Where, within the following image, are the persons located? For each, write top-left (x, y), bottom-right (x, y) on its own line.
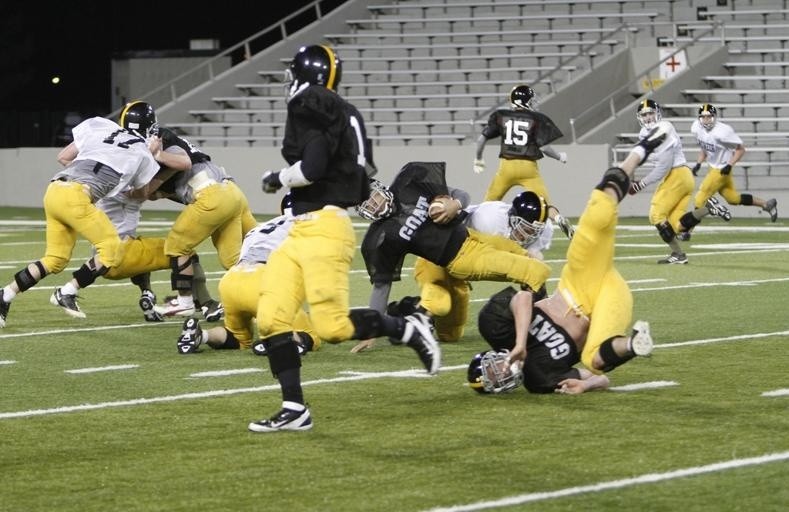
top-left (627, 97), bottom-right (731, 266)
top-left (248, 44), bottom-right (440, 433)
top-left (0, 101), bottom-right (258, 329)
top-left (350, 160), bottom-right (552, 354)
top-left (390, 193), bottom-right (574, 347)
top-left (468, 121), bottom-right (673, 394)
top-left (675, 104), bottom-right (778, 242)
top-left (176, 195), bottom-right (321, 354)
top-left (472, 85), bottom-right (577, 240)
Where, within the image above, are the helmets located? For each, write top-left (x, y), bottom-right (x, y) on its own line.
top-left (635, 99), bottom-right (662, 130)
top-left (697, 104), bottom-right (717, 131)
top-left (353, 179), bottom-right (394, 222)
top-left (506, 191), bottom-right (548, 248)
top-left (280, 191), bottom-right (292, 215)
top-left (509, 85), bottom-right (540, 112)
top-left (467, 347), bottom-right (524, 394)
top-left (281, 45), bottom-right (342, 106)
top-left (117, 99), bottom-right (159, 141)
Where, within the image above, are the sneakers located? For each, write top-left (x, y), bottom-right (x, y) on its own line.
top-left (632, 124), bottom-right (670, 166)
top-left (396, 313), bottom-right (442, 375)
top-left (0, 287), bottom-right (11, 327)
top-left (253, 340), bottom-right (308, 357)
top-left (707, 197), bottom-right (731, 221)
top-left (139, 289), bottom-right (223, 355)
top-left (630, 320), bottom-right (653, 357)
top-left (558, 216), bottom-right (573, 238)
top-left (248, 400), bottom-right (313, 433)
top-left (50, 288), bottom-right (85, 317)
top-left (763, 198), bottom-right (777, 222)
top-left (657, 228), bottom-right (692, 265)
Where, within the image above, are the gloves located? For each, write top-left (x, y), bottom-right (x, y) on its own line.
top-left (720, 165), bottom-right (732, 175)
top-left (558, 152), bottom-right (567, 163)
top-left (693, 164), bottom-right (701, 176)
top-left (628, 182), bottom-right (641, 195)
top-left (473, 159), bottom-right (485, 173)
top-left (262, 170), bottom-right (282, 193)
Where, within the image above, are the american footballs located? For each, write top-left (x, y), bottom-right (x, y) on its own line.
top-left (429, 196), bottom-right (457, 223)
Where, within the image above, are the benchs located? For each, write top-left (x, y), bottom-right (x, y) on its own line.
top-left (165, 0), bottom-right (789, 190)
